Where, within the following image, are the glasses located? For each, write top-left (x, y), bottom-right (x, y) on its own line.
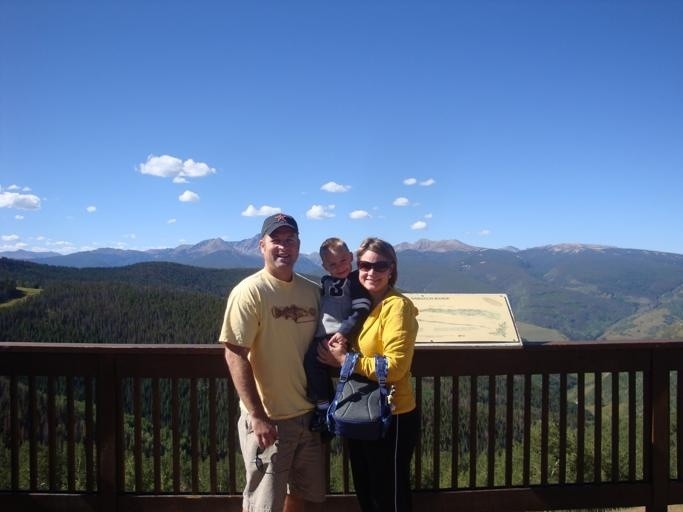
top-left (255, 438), bottom-right (289, 476)
top-left (357, 261), bottom-right (393, 273)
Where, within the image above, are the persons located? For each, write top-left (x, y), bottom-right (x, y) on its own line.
top-left (219, 213), bottom-right (328, 512)
top-left (316, 235), bottom-right (419, 512)
top-left (302, 237), bottom-right (374, 431)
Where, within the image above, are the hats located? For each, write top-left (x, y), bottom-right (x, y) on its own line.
top-left (262, 213), bottom-right (298, 236)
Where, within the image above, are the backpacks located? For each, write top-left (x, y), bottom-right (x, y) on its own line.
top-left (325, 352), bottom-right (393, 441)
